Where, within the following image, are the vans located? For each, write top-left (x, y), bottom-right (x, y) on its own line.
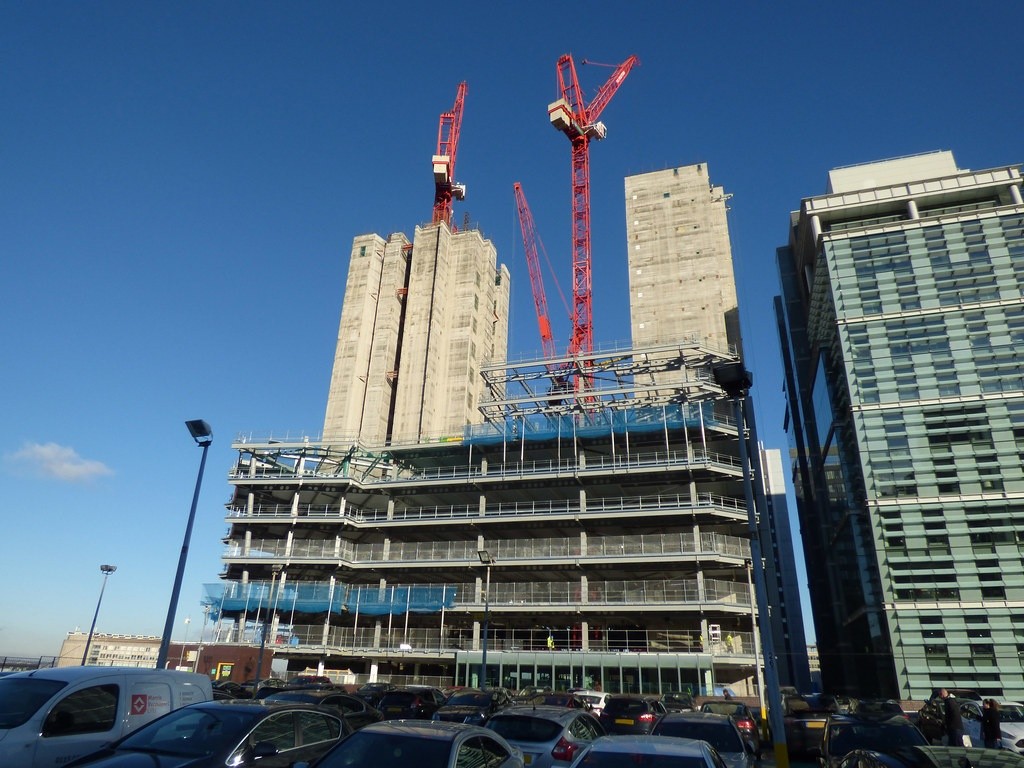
top-left (0, 665), bottom-right (213, 768)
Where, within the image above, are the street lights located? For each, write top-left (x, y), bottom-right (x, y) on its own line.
top-left (476, 550), bottom-right (496, 692)
top-left (710, 362), bottom-right (790, 768)
top-left (156, 419), bottom-right (213, 669)
top-left (81, 564), bottom-right (118, 666)
top-left (252, 564), bottom-right (284, 695)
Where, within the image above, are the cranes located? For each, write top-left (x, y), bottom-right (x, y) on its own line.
top-left (511, 53), bottom-right (642, 427)
top-left (430, 80), bottom-right (468, 229)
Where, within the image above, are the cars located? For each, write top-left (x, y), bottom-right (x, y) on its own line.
top-left (766, 688), bottom-right (1024, 768)
top-left (57, 674), bottom-right (770, 768)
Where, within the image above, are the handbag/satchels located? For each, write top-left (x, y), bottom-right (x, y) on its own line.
top-left (962, 734), bottom-right (973, 748)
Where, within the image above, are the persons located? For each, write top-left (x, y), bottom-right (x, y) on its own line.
top-left (939, 689), bottom-right (964, 747)
top-left (980, 698), bottom-right (1002, 749)
top-left (594, 681), bottom-right (601, 692)
top-left (915, 718), bottom-right (943, 745)
top-left (723, 689), bottom-right (731, 700)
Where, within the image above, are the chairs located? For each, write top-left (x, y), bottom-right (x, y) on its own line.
top-left (207, 715), bottom-right (244, 747)
top-left (704, 706), bottom-right (713, 713)
top-left (735, 707), bottom-right (744, 715)
